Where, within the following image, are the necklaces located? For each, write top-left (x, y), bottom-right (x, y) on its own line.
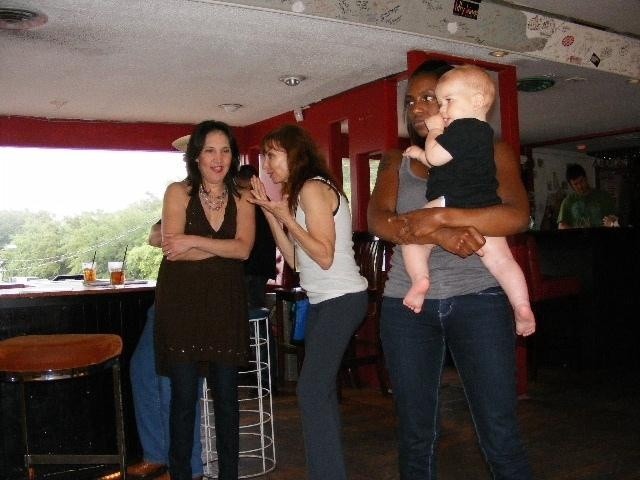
top-left (199, 185), bottom-right (228, 211)
top-left (424, 166), bottom-right (431, 176)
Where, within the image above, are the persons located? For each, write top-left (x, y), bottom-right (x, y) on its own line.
top-left (238, 163), bottom-right (271, 313)
top-left (246, 123), bottom-right (369, 480)
top-left (126, 132), bottom-right (202, 480)
top-left (366, 60), bottom-right (536, 480)
top-left (401, 65), bottom-right (538, 338)
top-left (157, 118), bottom-right (257, 480)
top-left (555, 164), bottom-right (616, 230)
top-left (601, 213), bottom-right (640, 228)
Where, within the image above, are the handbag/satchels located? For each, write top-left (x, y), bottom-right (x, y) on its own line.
top-left (289, 299), bottom-right (309, 347)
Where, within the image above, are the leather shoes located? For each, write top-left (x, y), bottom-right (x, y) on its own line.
top-left (126, 460), bottom-right (171, 480)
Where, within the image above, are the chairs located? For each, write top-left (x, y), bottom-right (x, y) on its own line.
top-left (274, 231), bottom-right (383, 406)
top-left (519, 235), bottom-right (583, 383)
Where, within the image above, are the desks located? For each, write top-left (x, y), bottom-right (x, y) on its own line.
top-left (1, 280), bottom-right (156, 478)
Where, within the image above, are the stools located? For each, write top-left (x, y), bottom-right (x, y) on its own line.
top-left (0, 332), bottom-right (126, 479)
top-left (196, 302), bottom-right (278, 479)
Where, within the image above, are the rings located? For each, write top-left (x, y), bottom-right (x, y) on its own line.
top-left (167, 252), bottom-right (172, 255)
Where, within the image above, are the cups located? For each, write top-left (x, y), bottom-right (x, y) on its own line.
top-left (108, 261), bottom-right (123, 285)
top-left (82, 263), bottom-right (97, 284)
